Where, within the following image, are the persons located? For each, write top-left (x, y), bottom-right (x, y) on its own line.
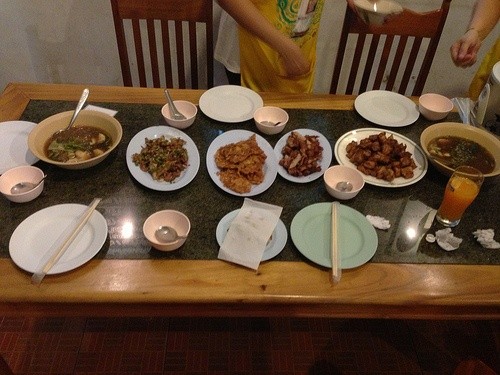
top-left (451, 0), bottom-right (500, 97)
top-left (214, 0), bottom-right (376, 91)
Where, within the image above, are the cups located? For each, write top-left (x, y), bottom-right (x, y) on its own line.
top-left (435, 167), bottom-right (484, 227)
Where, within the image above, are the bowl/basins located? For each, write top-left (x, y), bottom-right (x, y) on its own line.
top-left (421, 123), bottom-right (500, 177)
top-left (161, 100), bottom-right (197, 129)
top-left (418, 93), bottom-right (453, 120)
top-left (142, 209), bottom-right (190, 252)
top-left (0, 166), bottom-right (44, 203)
top-left (324, 164), bottom-right (365, 200)
top-left (28, 109), bottom-right (122, 168)
top-left (254, 105), bottom-right (288, 135)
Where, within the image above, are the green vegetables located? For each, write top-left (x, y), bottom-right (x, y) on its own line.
top-left (48, 131), bottom-right (112, 160)
top-left (149, 147), bottom-right (188, 173)
top-left (448, 138), bottom-right (482, 168)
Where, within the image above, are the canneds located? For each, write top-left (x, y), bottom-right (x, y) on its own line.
top-left (470, 60), bottom-right (500, 139)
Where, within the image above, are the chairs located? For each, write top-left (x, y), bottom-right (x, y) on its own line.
top-left (110, 0), bottom-right (214, 90)
top-left (328, 0), bottom-right (452, 96)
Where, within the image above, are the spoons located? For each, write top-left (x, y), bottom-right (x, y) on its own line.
top-left (164, 89), bottom-right (186, 120)
top-left (155, 226), bottom-right (187, 242)
top-left (261, 120), bottom-right (281, 126)
top-left (10, 175), bottom-right (47, 194)
top-left (336, 181), bottom-right (353, 192)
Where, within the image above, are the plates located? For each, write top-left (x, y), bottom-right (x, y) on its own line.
top-left (8, 203), bottom-right (108, 274)
top-left (126, 125), bottom-right (200, 191)
top-left (353, 0), bottom-right (402, 23)
top-left (0, 120), bottom-right (40, 174)
top-left (199, 85), bottom-right (263, 123)
top-left (206, 90), bottom-right (428, 269)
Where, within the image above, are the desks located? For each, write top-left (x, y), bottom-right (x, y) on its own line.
top-left (0, 82), bottom-right (500, 375)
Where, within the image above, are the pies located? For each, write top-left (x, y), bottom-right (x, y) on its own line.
top-left (215, 133), bottom-right (267, 193)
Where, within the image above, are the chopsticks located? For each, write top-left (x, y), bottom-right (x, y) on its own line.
top-left (331, 202), bottom-right (338, 277)
top-left (42, 198), bottom-right (100, 272)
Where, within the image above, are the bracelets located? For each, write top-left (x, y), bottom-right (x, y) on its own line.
top-left (464, 28), bottom-right (480, 37)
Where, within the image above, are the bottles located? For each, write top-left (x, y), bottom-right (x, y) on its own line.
top-left (472, 61), bottom-right (500, 134)
